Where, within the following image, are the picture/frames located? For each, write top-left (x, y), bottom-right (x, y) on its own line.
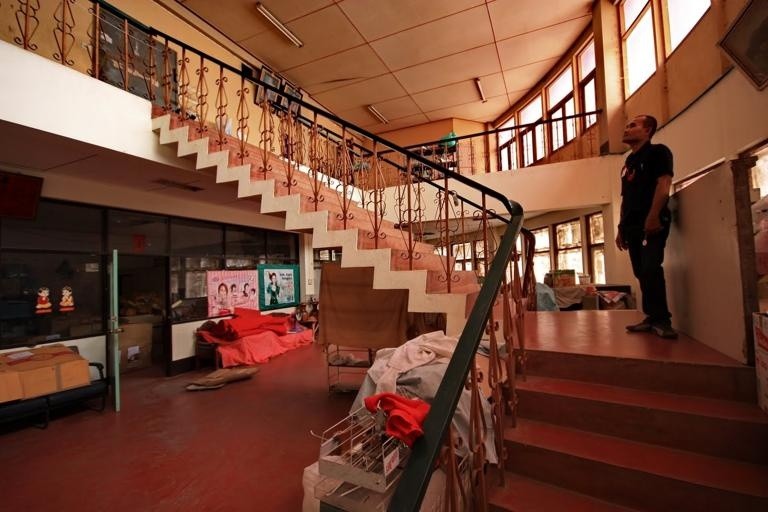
top-left (255, 66), bottom-right (303, 125)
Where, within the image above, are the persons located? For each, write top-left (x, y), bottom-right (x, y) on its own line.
top-left (615, 114), bottom-right (679, 339)
top-left (267, 273), bottom-right (280, 305)
top-left (213, 282), bottom-right (257, 313)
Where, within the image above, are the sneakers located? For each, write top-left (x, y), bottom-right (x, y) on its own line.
top-left (650, 324), bottom-right (678, 339)
top-left (626, 322), bottom-right (654, 332)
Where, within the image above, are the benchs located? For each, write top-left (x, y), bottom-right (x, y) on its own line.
top-left (1, 345), bottom-right (108, 430)
